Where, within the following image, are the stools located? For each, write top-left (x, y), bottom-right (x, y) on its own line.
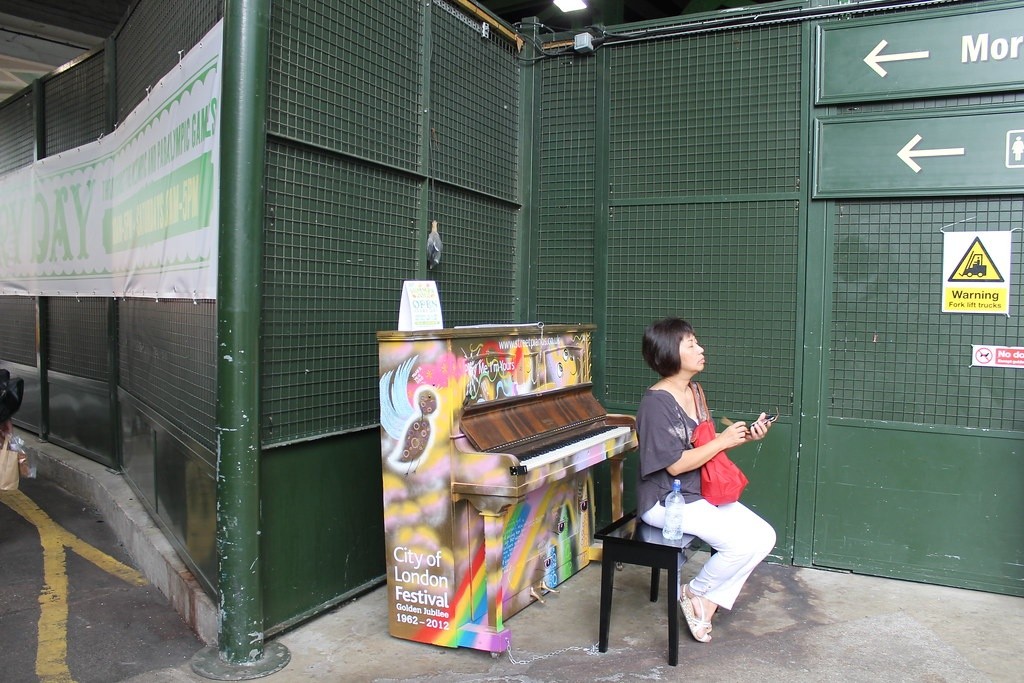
top-left (593, 509), bottom-right (718, 666)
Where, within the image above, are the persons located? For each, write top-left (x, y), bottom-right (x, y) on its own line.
top-left (0, 368), bottom-right (24, 450)
top-left (634, 316), bottom-right (776, 641)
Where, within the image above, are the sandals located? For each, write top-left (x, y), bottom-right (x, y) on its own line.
top-left (680, 583), bottom-right (712, 643)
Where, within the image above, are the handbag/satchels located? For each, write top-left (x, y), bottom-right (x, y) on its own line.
top-left (687, 382), bottom-right (748, 507)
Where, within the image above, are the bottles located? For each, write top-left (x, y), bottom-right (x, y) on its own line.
top-left (663, 480), bottom-right (685, 540)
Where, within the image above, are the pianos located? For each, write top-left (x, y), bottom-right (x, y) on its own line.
top-left (375, 321), bottom-right (639, 660)
